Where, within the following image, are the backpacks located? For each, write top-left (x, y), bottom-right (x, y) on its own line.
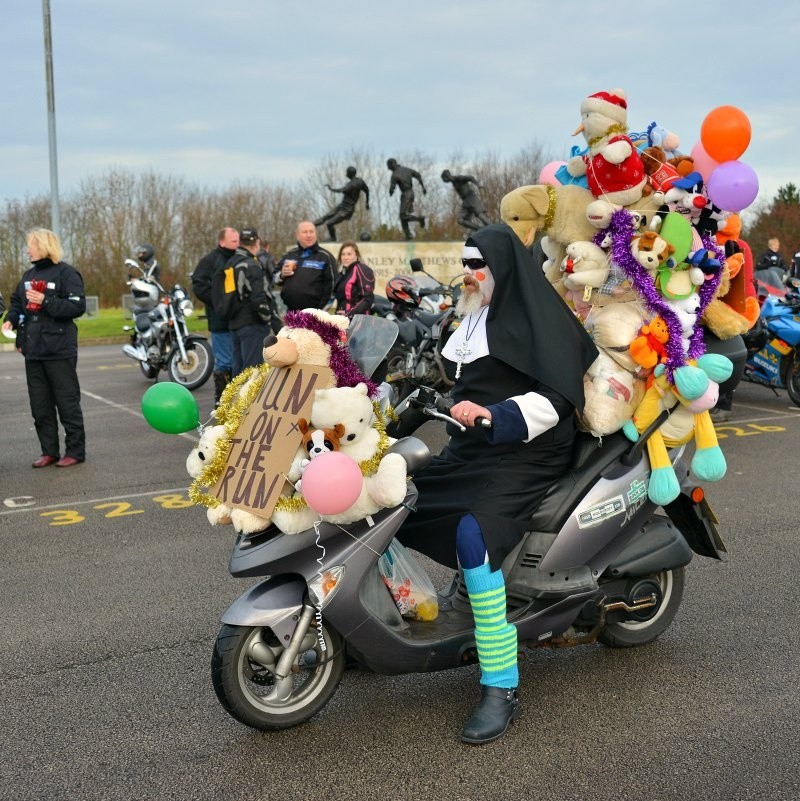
top-left (212, 255), bottom-right (250, 320)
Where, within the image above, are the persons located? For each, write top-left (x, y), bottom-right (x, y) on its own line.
top-left (311, 166), bottom-right (371, 243)
top-left (192, 228), bottom-right (241, 411)
top-left (386, 158), bottom-right (427, 241)
top-left (389, 222), bottom-right (584, 738)
top-left (441, 169), bottom-right (491, 231)
top-left (757, 239), bottom-right (788, 269)
top-left (259, 238), bottom-right (276, 285)
top-left (134, 243), bottom-right (162, 298)
top-left (270, 223), bottom-right (339, 315)
top-left (226, 227), bottom-right (272, 380)
top-left (1, 226), bottom-right (88, 467)
top-left (334, 241), bottom-right (375, 319)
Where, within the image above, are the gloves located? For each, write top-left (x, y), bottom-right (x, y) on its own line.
top-left (26, 278), bottom-right (47, 309)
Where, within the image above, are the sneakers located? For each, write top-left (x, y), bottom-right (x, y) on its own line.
top-left (56, 456), bottom-right (77, 466)
top-left (31, 454), bottom-right (60, 468)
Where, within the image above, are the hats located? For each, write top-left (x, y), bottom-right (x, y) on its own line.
top-left (240, 228), bottom-right (258, 240)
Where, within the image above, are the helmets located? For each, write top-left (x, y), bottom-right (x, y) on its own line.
top-left (137, 244), bottom-right (155, 261)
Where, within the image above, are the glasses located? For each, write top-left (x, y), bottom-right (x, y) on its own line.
top-left (461, 258), bottom-right (487, 270)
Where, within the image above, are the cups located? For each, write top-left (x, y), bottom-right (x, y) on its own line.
top-left (24, 280), bottom-right (38, 304)
top-left (284, 259), bottom-right (295, 276)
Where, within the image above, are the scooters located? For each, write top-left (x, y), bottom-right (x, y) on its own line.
top-left (320, 255), bottom-right (468, 402)
top-left (208, 310), bottom-right (745, 733)
top-left (747, 260), bottom-right (800, 410)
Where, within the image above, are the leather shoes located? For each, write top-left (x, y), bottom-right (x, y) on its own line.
top-left (460, 685), bottom-right (523, 743)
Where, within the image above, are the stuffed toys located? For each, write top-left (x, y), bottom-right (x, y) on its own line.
top-left (186, 305), bottom-right (409, 538)
top-left (492, 86), bottom-right (760, 508)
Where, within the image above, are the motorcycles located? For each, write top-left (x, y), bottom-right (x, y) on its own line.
top-left (122, 282), bottom-right (216, 391)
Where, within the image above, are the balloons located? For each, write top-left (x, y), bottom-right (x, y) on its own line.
top-left (688, 141), bottom-right (718, 186)
top-left (702, 105), bottom-right (752, 163)
top-left (298, 452), bottom-right (367, 521)
top-left (142, 381), bottom-right (203, 437)
top-left (537, 161), bottom-right (573, 188)
top-left (708, 164), bottom-right (758, 214)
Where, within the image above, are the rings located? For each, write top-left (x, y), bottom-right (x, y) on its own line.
top-left (462, 412), bottom-right (468, 417)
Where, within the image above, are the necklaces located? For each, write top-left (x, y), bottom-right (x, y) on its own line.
top-left (454, 305), bottom-right (487, 379)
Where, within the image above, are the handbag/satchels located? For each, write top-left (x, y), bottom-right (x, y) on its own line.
top-left (376, 540), bottom-right (439, 622)
